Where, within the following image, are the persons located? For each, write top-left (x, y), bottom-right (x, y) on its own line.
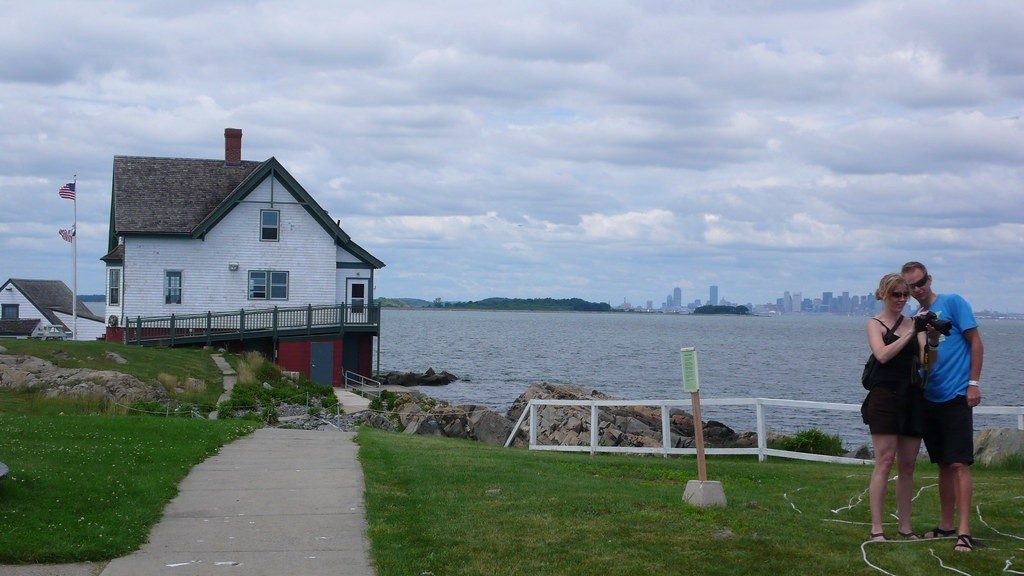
top-left (900, 261), bottom-right (984, 553)
top-left (860, 273), bottom-right (943, 542)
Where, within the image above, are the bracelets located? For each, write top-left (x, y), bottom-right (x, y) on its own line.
top-left (929, 342), bottom-right (939, 351)
top-left (969, 380), bottom-right (980, 387)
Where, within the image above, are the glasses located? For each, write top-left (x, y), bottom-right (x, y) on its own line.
top-left (889, 291), bottom-right (909, 298)
top-left (907, 274), bottom-right (929, 290)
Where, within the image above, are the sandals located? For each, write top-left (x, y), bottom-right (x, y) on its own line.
top-left (871, 532), bottom-right (888, 541)
top-left (898, 531), bottom-right (921, 540)
top-left (921, 527), bottom-right (956, 538)
top-left (953, 535), bottom-right (972, 552)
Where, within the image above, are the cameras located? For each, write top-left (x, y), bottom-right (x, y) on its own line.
top-left (914, 310), bottom-right (953, 337)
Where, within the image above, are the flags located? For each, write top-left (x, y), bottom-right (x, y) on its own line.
top-left (58, 224), bottom-right (76, 244)
top-left (59, 183), bottom-right (76, 200)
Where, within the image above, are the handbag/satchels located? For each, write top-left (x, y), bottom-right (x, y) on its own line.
top-left (862, 353), bottom-right (886, 390)
